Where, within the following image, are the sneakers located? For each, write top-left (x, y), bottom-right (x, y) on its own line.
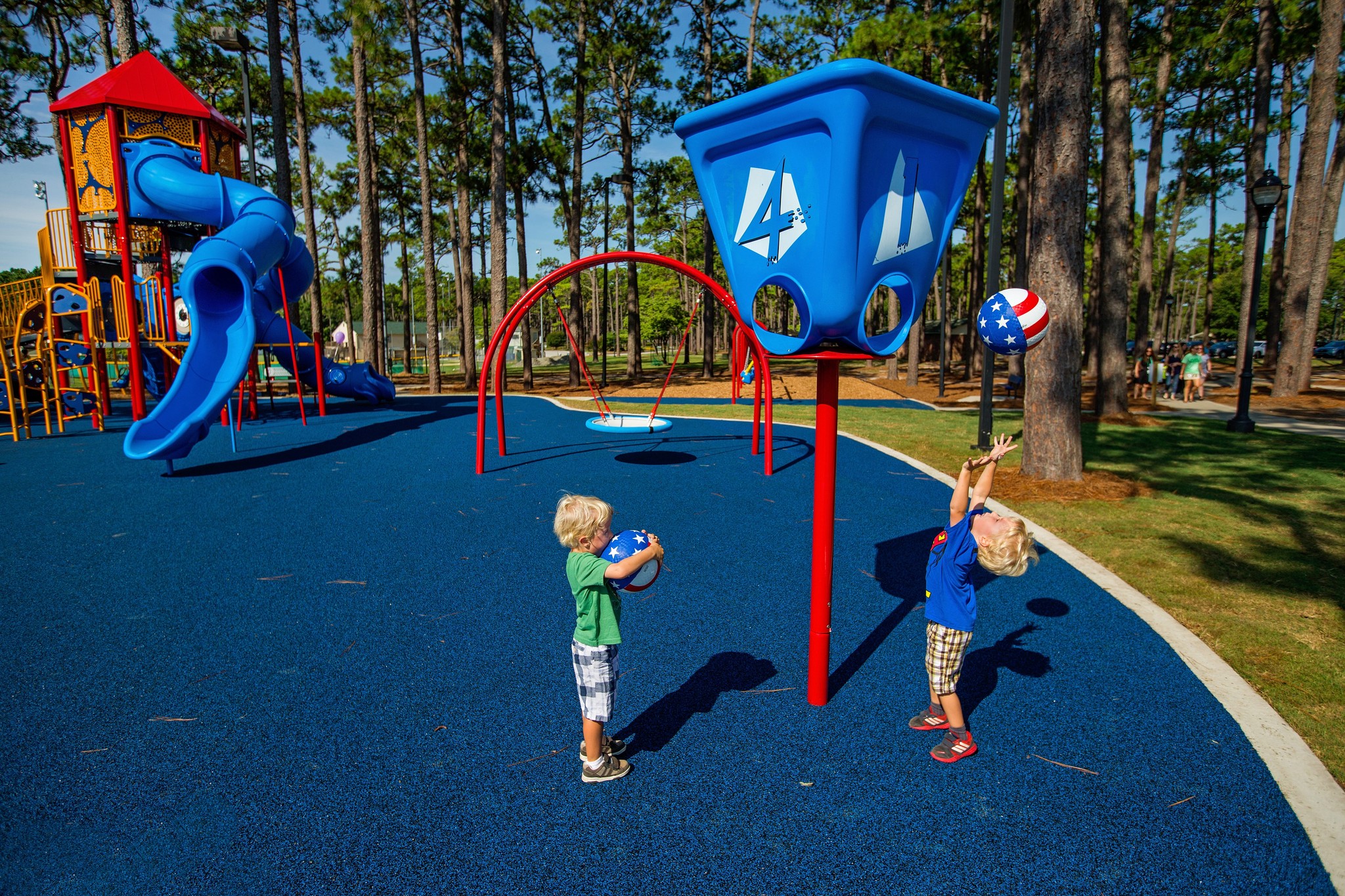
top-left (930, 731), bottom-right (977, 762)
top-left (908, 706), bottom-right (950, 730)
top-left (580, 735), bottom-right (627, 761)
top-left (581, 752), bottom-right (630, 783)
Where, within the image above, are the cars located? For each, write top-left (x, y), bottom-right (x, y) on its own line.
top-left (1125, 341), bottom-right (1134, 355)
top-left (1208, 341), bottom-right (1281, 359)
top-left (1313, 341), bottom-right (1345, 359)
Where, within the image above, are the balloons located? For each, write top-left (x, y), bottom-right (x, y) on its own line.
top-left (335, 332), bottom-right (345, 344)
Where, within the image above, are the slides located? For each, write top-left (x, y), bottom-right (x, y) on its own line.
top-left (250, 232), bottom-right (396, 400)
top-left (123, 145), bottom-right (296, 461)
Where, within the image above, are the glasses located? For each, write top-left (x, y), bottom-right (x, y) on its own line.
top-left (1193, 348), bottom-right (1199, 350)
top-left (1172, 346), bottom-right (1178, 348)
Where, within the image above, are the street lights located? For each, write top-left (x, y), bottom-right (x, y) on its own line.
top-left (536, 248), bottom-right (544, 359)
top-left (444, 272), bottom-right (453, 355)
top-left (33, 180), bottom-right (54, 256)
top-left (1227, 163), bottom-right (1292, 432)
top-left (438, 283), bottom-right (446, 348)
top-left (1162, 294), bottom-right (1174, 359)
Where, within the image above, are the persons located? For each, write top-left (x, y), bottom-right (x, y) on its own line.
top-left (553, 494), bottom-right (664, 783)
top-left (908, 434), bottom-right (1040, 763)
top-left (1131, 347), bottom-right (1154, 399)
top-left (1163, 343), bottom-right (1212, 403)
top-left (21, 349), bottom-right (30, 360)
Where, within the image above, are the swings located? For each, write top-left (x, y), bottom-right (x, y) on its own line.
top-left (547, 284), bottom-right (705, 436)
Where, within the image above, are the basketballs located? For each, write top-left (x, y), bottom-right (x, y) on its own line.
top-left (976, 289), bottom-right (1049, 356)
top-left (597, 530), bottom-right (661, 595)
